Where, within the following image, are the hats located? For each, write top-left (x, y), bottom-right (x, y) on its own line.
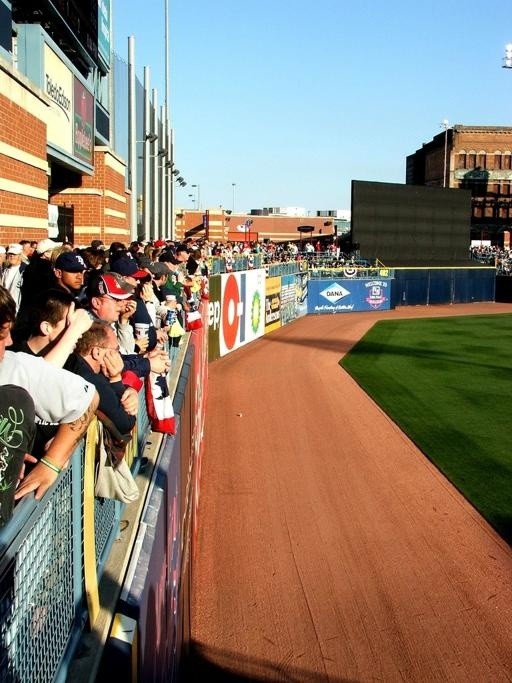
top-left (0, 240), bottom-right (195, 300)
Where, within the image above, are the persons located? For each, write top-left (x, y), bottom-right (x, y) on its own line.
top-left (0, 239), bottom-right (364, 683)
top-left (471, 245), bottom-right (512, 276)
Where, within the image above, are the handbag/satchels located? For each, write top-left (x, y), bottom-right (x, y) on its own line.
top-left (186, 311), bottom-right (202, 331)
top-left (145, 370), bottom-right (176, 436)
top-left (95, 446), bottom-right (140, 503)
top-left (168, 318), bottom-right (185, 338)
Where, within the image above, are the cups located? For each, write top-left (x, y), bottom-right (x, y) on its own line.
top-left (166, 294), bottom-right (176, 304)
top-left (166, 308), bottom-right (176, 325)
top-left (135, 321), bottom-right (149, 337)
